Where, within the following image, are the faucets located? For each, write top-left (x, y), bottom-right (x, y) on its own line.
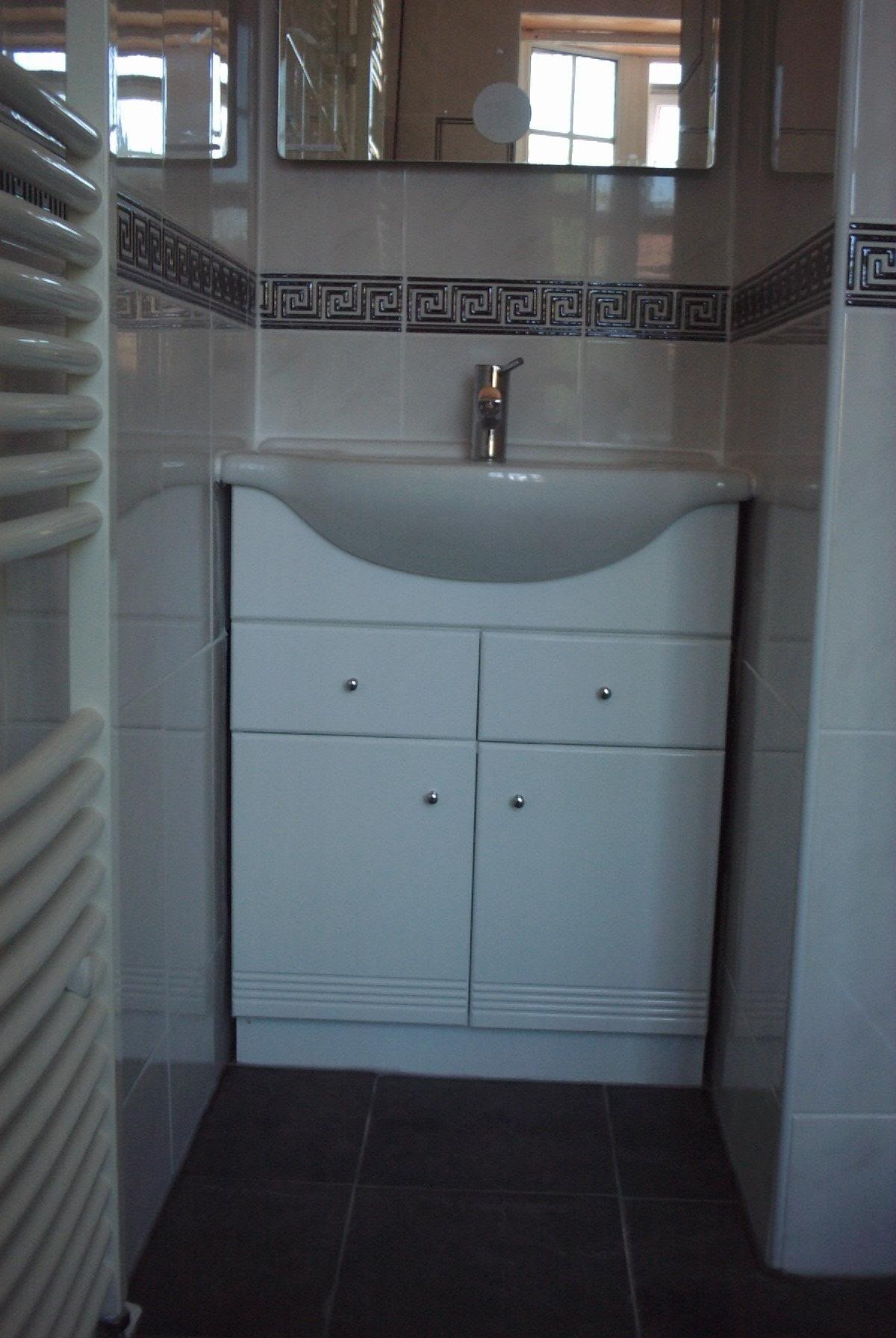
top-left (469, 357), bottom-right (526, 462)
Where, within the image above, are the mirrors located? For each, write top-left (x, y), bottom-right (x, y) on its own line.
top-left (273, 0), bottom-right (722, 173)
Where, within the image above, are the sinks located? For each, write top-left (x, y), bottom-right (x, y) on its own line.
top-left (222, 435), bottom-right (704, 480)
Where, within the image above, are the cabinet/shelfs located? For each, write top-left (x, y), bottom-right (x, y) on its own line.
top-left (226, 484), bottom-right (729, 1087)
